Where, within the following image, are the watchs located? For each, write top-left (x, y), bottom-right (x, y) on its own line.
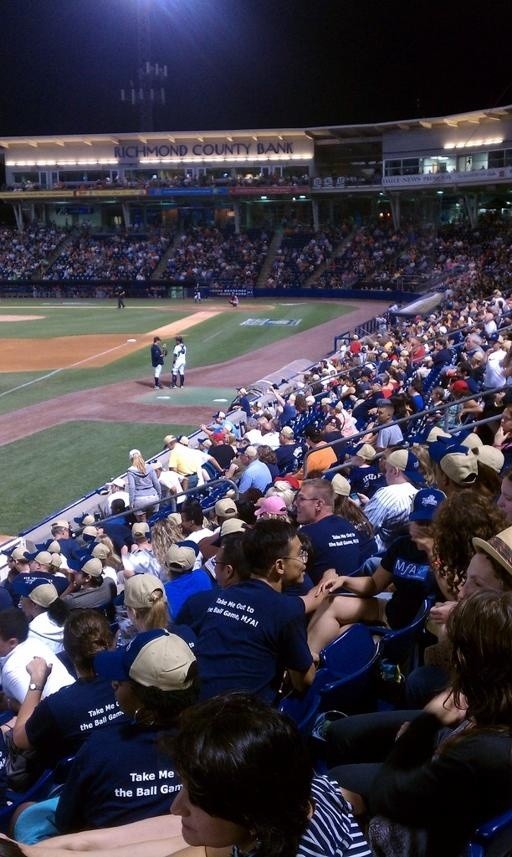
top-left (28, 684), bottom-right (43, 691)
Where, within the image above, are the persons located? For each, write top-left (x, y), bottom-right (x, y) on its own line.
top-left (253, 496), bottom-right (285, 524)
top-left (333, 590), bottom-right (512, 820)
top-left (168, 335), bottom-right (187, 388)
top-left (164, 513), bottom-right (185, 528)
top-left (145, 519), bottom-right (182, 576)
top-left (273, 223), bottom-right (461, 297)
top-left (46, 516), bottom-right (81, 568)
top-left (406, 428), bottom-right (449, 487)
top-left (268, 480), bottom-right (295, 517)
top-left (84, 522), bottom-right (136, 553)
top-left (37, 536), bottom-right (63, 559)
top-left (73, 223), bottom-right (273, 290)
top-left (302, 481), bottom-right (442, 671)
top-left (173, 432), bottom-right (224, 485)
top-left (257, 445), bottom-right (278, 481)
top-left (158, 691), bottom-right (376, 857)
top-left (10, 628), bottom-right (207, 848)
top-left (161, 433), bottom-right (200, 490)
top-left (11, 548), bottom-right (66, 600)
top-left (101, 480), bottom-right (130, 516)
top-left (185, 521), bottom-right (334, 714)
top-left (126, 446), bottom-right (162, 524)
top-left (0, 224), bottom-right (73, 291)
top-left (316, 524), bottom-right (512, 745)
top-left (82, 542), bottom-right (118, 591)
top-left (14, 610), bottom-right (126, 766)
top-left (288, 482), bottom-right (362, 581)
top-left (209, 496), bottom-right (236, 529)
top-left (68, 511), bottom-right (99, 530)
top-left (461, 219), bottom-right (512, 292)
top-left (235, 445), bottom-right (272, 499)
top-left (193, 294), bottom-right (512, 452)
top-left (1, 543), bottom-right (30, 581)
top-left (17, 573), bottom-right (73, 653)
top-left (114, 576), bottom-right (201, 682)
top-left (78, 523), bottom-right (98, 550)
top-left (423, 490), bottom-right (506, 595)
top-left (116, 285), bottom-right (127, 309)
top-left (498, 468), bottom-right (512, 528)
top-left (196, 520), bottom-right (250, 558)
top-left (10, 172), bottom-right (361, 193)
top-left (158, 549), bottom-right (214, 625)
top-left (175, 499), bottom-right (212, 548)
top-left (464, 441), bottom-right (503, 499)
top-left (94, 534), bottom-right (122, 569)
top-left (101, 497), bottom-right (134, 530)
top-left (328, 474), bottom-right (375, 536)
top-left (361, 445), bottom-right (420, 553)
top-left (120, 523), bottom-right (161, 576)
top-left (0, 607), bottom-right (78, 738)
top-left (59, 550), bottom-right (118, 631)
top-left (338, 440), bottom-right (377, 493)
top-left (432, 449), bottom-right (474, 492)
top-left (46, 551), bottom-right (75, 595)
top-left (147, 456), bottom-right (189, 507)
top-left (172, 534), bottom-right (247, 644)
top-left (151, 336), bottom-right (166, 389)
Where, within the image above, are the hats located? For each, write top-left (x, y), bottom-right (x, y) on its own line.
top-left (282, 379), bottom-right (288, 383)
top-left (34, 551), bottom-right (52, 564)
top-left (112, 574), bottom-right (168, 608)
top-left (215, 497), bottom-right (238, 518)
top-left (281, 426), bottom-right (294, 438)
top-left (73, 513), bottom-right (95, 526)
top-left (212, 411), bottom-right (226, 418)
top-left (161, 435), bottom-right (176, 449)
top-left (328, 400), bottom-right (343, 411)
top-left (471, 527), bottom-right (512, 574)
top-left (105, 478), bottom-right (126, 489)
top-left (344, 441), bottom-right (376, 461)
top-left (386, 449), bottom-right (426, 483)
top-left (154, 337), bottom-right (161, 341)
top-left (92, 628), bottom-right (197, 690)
top-left (486, 332), bottom-right (503, 344)
top-left (321, 471), bottom-right (351, 498)
top-left (169, 511), bottom-right (183, 525)
top-left (237, 446), bottom-right (258, 458)
top-left (82, 525), bottom-right (97, 538)
top-left (50, 519), bottom-right (73, 537)
top-left (302, 427), bottom-right (320, 437)
top-left (409, 488), bottom-right (448, 523)
top-left (164, 540), bottom-right (200, 574)
top-left (35, 539), bottom-right (60, 554)
top-left (177, 434), bottom-right (190, 446)
top-left (322, 416), bottom-right (341, 426)
top-left (198, 438), bottom-right (213, 448)
top-left (254, 496), bottom-right (288, 517)
top-left (149, 459), bottom-right (162, 470)
top-left (449, 380), bottom-right (469, 391)
top-left (220, 518), bottom-right (247, 536)
top-left (2, 545), bottom-right (16, 559)
top-left (473, 442), bottom-right (506, 474)
top-left (11, 572), bottom-right (59, 608)
top-left (236, 386), bottom-right (246, 395)
top-left (76, 542), bottom-right (110, 560)
top-left (131, 522), bottom-right (150, 539)
top-left (270, 384), bottom-right (279, 390)
top-left (309, 368), bottom-right (318, 374)
top-left (437, 427), bottom-right (483, 451)
top-left (235, 438), bottom-right (250, 448)
top-left (128, 449), bottom-right (141, 457)
top-left (24, 549), bottom-right (37, 560)
top-left (429, 442), bottom-right (478, 487)
top-left (174, 336), bottom-right (182, 340)
top-left (49, 553), bottom-right (62, 566)
top-left (67, 556), bottom-right (103, 577)
top-left (9, 548), bottom-right (29, 561)
top-left (409, 425), bottom-right (446, 444)
top-left (466, 351), bottom-right (484, 363)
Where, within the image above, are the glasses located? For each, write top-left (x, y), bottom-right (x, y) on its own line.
top-left (299, 496), bottom-right (319, 502)
top-left (282, 550), bottom-right (309, 563)
top-left (211, 558), bottom-right (225, 565)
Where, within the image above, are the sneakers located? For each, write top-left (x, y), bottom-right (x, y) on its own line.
top-left (153, 384), bottom-right (163, 389)
top-left (180, 385), bottom-right (184, 389)
top-left (168, 384), bottom-right (176, 388)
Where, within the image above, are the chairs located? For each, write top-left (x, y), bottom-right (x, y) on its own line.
top-left (276, 686), bottom-right (321, 732)
top-left (315, 623), bottom-right (385, 700)
top-left (146, 503), bottom-right (172, 528)
top-left (419, 354), bottom-right (455, 406)
top-left (449, 837), bottom-right (489, 857)
top-left (481, 802), bottom-right (512, 857)
top-left (282, 399), bottom-right (324, 445)
top-left (367, 595), bottom-right (436, 658)
top-left (192, 465), bottom-right (244, 515)
